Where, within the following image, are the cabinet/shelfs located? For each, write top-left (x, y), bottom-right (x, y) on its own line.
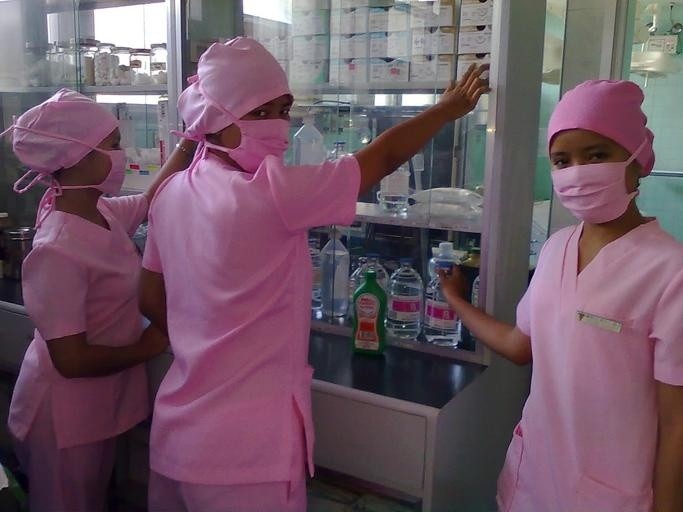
top-left (0, 0), bottom-right (174, 511)
top-left (175, 1), bottom-right (546, 512)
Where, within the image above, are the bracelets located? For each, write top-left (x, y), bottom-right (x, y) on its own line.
top-left (175, 144), bottom-right (192, 156)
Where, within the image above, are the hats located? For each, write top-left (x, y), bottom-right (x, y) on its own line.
top-left (177, 37), bottom-right (293, 138)
top-left (13, 88), bottom-right (120, 175)
top-left (548, 80), bottom-right (656, 177)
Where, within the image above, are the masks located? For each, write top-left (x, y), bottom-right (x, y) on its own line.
top-left (551, 138), bottom-right (651, 223)
top-left (0, 115), bottom-right (126, 230)
top-left (168, 75), bottom-right (290, 175)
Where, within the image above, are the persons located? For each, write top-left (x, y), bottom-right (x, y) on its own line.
top-left (0, 87), bottom-right (197, 511)
top-left (137, 37), bottom-right (491, 511)
top-left (436, 80), bottom-right (683, 511)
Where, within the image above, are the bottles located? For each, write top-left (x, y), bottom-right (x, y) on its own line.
top-left (319, 229), bottom-right (349, 317)
top-left (380, 161), bottom-right (407, 211)
top-left (424, 242), bottom-right (480, 347)
top-left (292, 113), bottom-right (346, 169)
top-left (386, 260), bottom-right (423, 338)
top-left (350, 253), bottom-right (389, 312)
top-left (306, 239), bottom-right (321, 319)
